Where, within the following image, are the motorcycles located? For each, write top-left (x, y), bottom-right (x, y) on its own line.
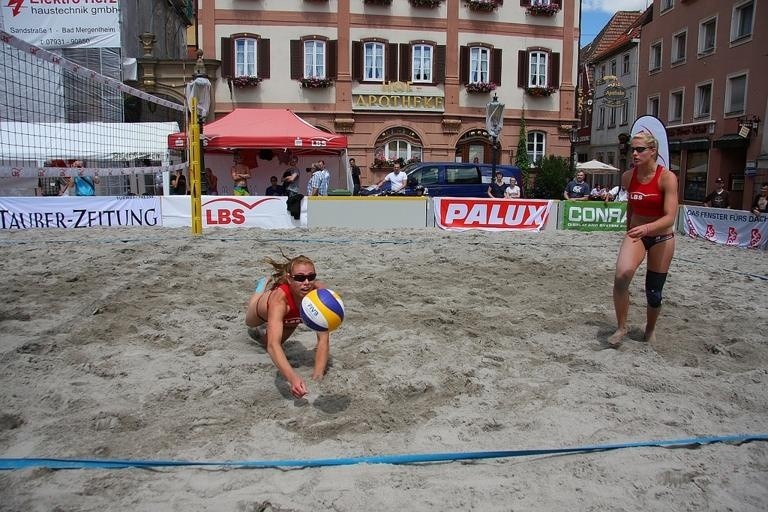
top-left (378, 188), bottom-right (397, 196)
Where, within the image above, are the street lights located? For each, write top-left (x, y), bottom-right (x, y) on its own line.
top-left (486, 93), bottom-right (505, 184)
top-left (184, 50), bottom-right (211, 195)
top-left (568, 125), bottom-right (580, 182)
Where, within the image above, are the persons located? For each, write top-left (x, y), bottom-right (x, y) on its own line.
top-left (36, 160), bottom-right (68, 196)
top-left (246, 244), bottom-right (330, 399)
top-left (487, 172), bottom-right (505, 198)
top-left (606, 128), bottom-right (678, 347)
top-left (349, 158), bottom-right (364, 196)
top-left (563, 172), bottom-right (590, 202)
top-left (231, 156), bottom-right (251, 196)
top-left (170, 165), bottom-right (186, 194)
top-left (68, 159), bottom-right (100, 196)
top-left (505, 177), bottom-right (520, 199)
top-left (204, 168), bottom-right (218, 194)
top-left (266, 156), bottom-right (330, 196)
top-left (589, 184), bottom-right (627, 202)
top-left (374, 162), bottom-right (406, 195)
top-left (703, 177), bottom-right (732, 209)
top-left (749, 183), bottom-right (768, 216)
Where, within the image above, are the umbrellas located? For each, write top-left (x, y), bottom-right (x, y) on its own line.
top-left (575, 159), bottom-right (621, 190)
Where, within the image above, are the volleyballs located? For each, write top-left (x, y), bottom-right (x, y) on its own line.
top-left (300, 289), bottom-right (344, 331)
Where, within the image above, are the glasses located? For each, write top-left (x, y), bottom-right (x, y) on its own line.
top-left (289, 274), bottom-right (315, 282)
top-left (630, 147), bottom-right (649, 153)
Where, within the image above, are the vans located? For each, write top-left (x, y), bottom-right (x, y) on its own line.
top-left (364, 162), bottom-right (524, 199)
